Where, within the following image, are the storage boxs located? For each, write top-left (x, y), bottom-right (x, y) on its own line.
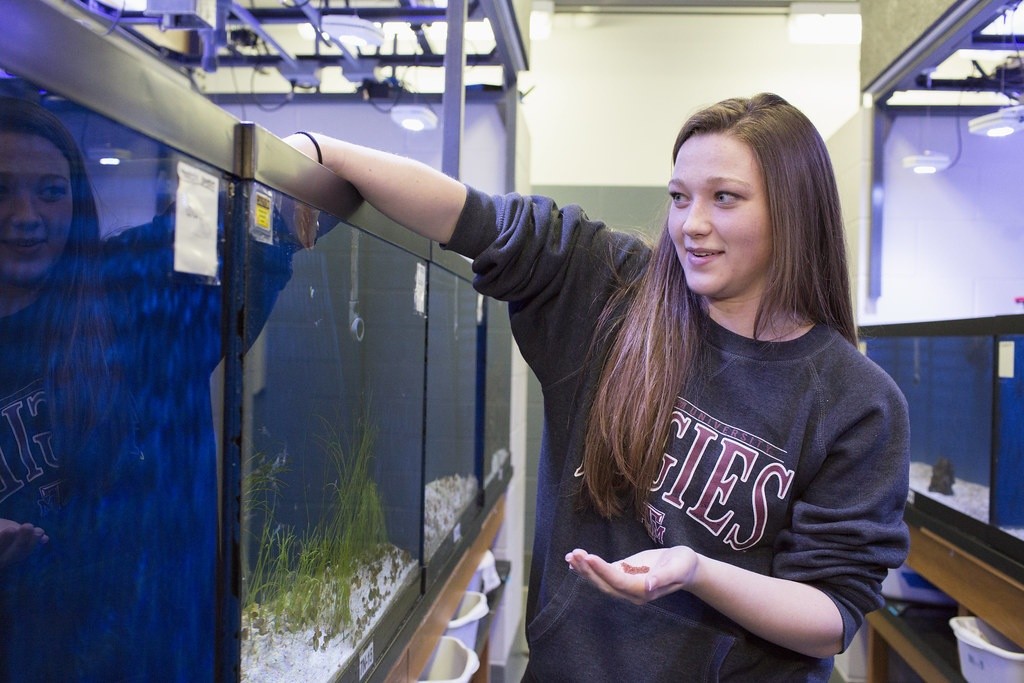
top-left (858, 312), bottom-right (1024, 586)
top-left (0, 1), bottom-right (514, 683)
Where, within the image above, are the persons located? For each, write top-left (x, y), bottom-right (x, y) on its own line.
top-left (280, 92), bottom-right (910, 683)
top-left (0, 95), bottom-right (338, 683)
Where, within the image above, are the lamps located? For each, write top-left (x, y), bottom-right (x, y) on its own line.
top-left (390, 104), bottom-right (439, 132)
top-left (965, 3), bottom-right (1024, 139)
top-left (901, 68), bottom-right (951, 174)
top-left (320, 11), bottom-right (384, 48)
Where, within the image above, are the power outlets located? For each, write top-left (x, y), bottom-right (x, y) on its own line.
top-left (340, 59), bottom-right (377, 82)
top-left (279, 60), bottom-right (323, 86)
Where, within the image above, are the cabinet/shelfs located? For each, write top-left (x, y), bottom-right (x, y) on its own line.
top-left (377, 491), bottom-right (514, 683)
top-left (865, 518), bottom-right (1024, 683)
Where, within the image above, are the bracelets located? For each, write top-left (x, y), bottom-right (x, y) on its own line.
top-left (295, 131), bottom-right (322, 164)
